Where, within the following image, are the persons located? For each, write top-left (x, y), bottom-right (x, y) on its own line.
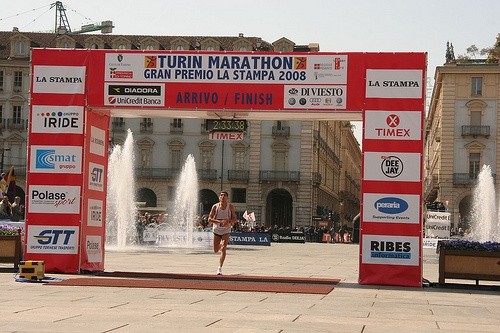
top-left (135, 212), bottom-right (353, 243)
top-left (208, 191), bottom-right (237, 275)
top-left (0, 173), bottom-right (25, 221)
top-left (439, 202), bottom-right (445, 212)
top-left (451, 228), bottom-right (471, 237)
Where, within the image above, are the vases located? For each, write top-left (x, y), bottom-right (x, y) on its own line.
top-left (439, 246), bottom-right (500, 285)
top-left (0, 235), bottom-right (23, 272)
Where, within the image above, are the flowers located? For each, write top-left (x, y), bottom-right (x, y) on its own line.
top-left (444, 239), bottom-right (500, 252)
top-left (0, 224), bottom-right (25, 238)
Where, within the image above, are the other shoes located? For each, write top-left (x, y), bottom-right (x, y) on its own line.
top-left (218, 268), bottom-right (222, 275)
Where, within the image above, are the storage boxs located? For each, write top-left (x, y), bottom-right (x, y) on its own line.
top-left (19, 260), bottom-right (45, 280)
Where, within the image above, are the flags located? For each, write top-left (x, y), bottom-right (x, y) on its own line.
top-left (0, 165), bottom-right (15, 204)
